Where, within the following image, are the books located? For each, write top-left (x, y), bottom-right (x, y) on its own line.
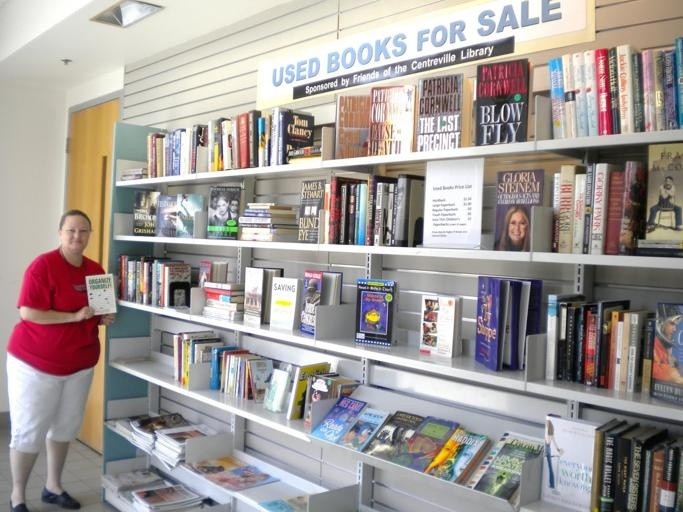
top-left (315, 395), bottom-right (543, 501)
top-left (475, 275), bottom-right (682, 406)
top-left (327, 166), bottom-right (543, 252)
top-left (333, 37), bottom-right (682, 159)
top-left (84, 273), bottom-right (116, 316)
top-left (540, 412), bottom-right (681, 512)
top-left (118, 106), bottom-right (321, 180)
top-left (549, 142), bottom-right (683, 258)
top-left (354, 277), bottom-right (398, 350)
top-left (104, 330), bottom-right (355, 511)
top-left (121, 255), bottom-right (348, 336)
top-left (421, 294), bottom-right (462, 356)
top-left (133, 180), bottom-right (327, 245)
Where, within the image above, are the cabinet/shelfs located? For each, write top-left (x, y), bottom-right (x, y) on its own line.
top-left (101, 63), bottom-right (682, 512)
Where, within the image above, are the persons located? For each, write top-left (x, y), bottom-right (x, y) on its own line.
top-left (2, 210), bottom-right (114, 511)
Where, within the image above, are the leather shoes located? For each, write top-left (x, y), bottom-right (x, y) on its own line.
top-left (9, 500), bottom-right (29, 512)
top-left (42, 487), bottom-right (81, 509)
